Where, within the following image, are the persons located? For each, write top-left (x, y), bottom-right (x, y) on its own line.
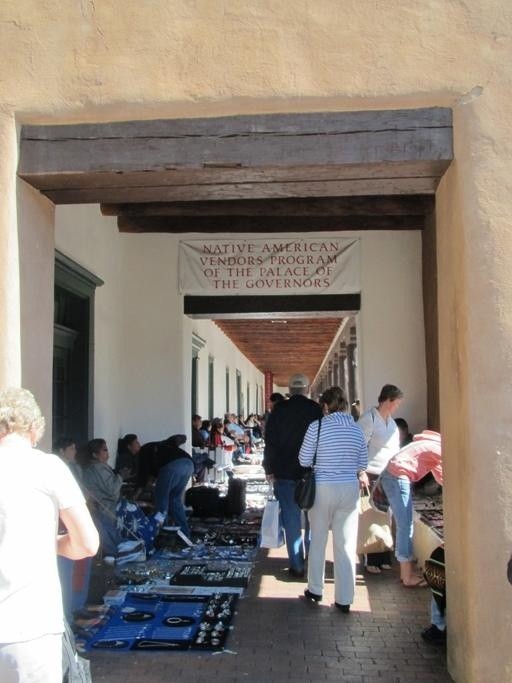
top-left (192, 413), bottom-right (265, 465)
top-left (393, 418), bottom-right (413, 447)
top-left (355, 383), bottom-right (404, 574)
top-left (263, 374), bottom-right (324, 578)
top-left (1, 386), bottom-right (100, 683)
top-left (51, 433), bottom-right (194, 553)
top-left (381, 429), bottom-right (444, 588)
top-left (298, 387), bottom-right (369, 612)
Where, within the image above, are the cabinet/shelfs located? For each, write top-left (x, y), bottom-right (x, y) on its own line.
top-left (407, 492), bottom-right (444, 575)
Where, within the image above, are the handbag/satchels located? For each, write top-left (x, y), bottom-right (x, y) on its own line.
top-left (357, 496), bottom-right (393, 554)
top-left (261, 500), bottom-right (284, 548)
top-left (297, 470), bottom-right (315, 509)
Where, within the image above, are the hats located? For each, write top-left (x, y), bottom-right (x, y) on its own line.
top-left (289, 374), bottom-right (309, 388)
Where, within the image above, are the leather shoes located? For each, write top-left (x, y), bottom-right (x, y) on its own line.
top-left (304, 590), bottom-right (321, 601)
top-left (335, 603), bottom-right (350, 613)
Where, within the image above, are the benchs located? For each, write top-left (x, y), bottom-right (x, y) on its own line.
top-left (191, 445), bottom-right (232, 484)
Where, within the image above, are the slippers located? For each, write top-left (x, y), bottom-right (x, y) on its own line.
top-left (401, 578), bottom-right (429, 588)
